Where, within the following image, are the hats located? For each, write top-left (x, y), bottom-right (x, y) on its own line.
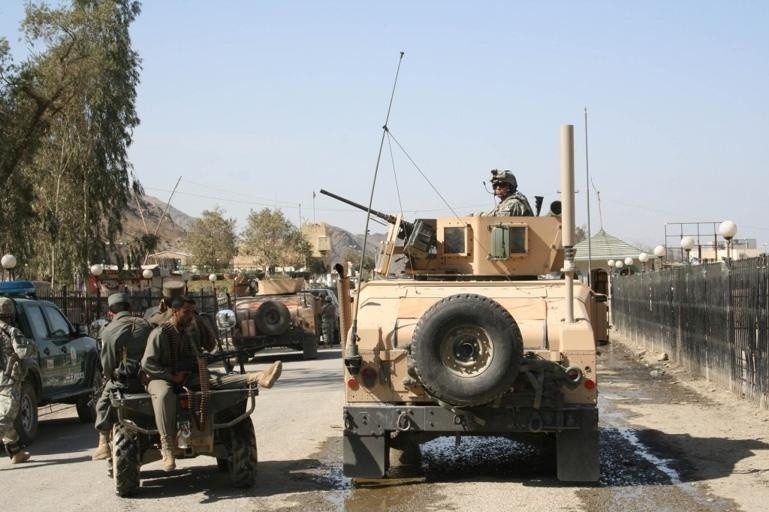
top-left (107, 293), bottom-right (131, 307)
top-left (162, 280), bottom-right (186, 298)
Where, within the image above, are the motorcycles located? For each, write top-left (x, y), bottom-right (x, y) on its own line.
top-left (108, 373), bottom-right (261, 496)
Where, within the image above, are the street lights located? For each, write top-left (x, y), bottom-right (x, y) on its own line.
top-left (720, 221), bottom-right (737, 258)
top-left (143, 270), bottom-right (153, 308)
top-left (639, 253), bottom-right (649, 272)
top-left (1, 254), bottom-right (17, 282)
top-left (182, 272), bottom-right (190, 296)
top-left (655, 245), bottom-right (666, 269)
top-left (681, 236), bottom-right (694, 263)
top-left (91, 264), bottom-right (103, 319)
top-left (608, 258), bottom-right (633, 276)
top-left (209, 274), bottom-right (217, 296)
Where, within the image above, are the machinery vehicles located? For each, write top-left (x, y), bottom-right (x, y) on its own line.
top-left (231, 278), bottom-right (322, 363)
top-left (319, 124), bottom-right (611, 482)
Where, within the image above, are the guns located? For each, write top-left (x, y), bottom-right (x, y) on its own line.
top-left (535, 196), bottom-right (544, 216)
top-left (141, 344), bottom-right (273, 392)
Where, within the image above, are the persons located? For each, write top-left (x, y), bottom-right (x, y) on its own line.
top-left (139, 294), bottom-right (282, 471)
top-left (464, 169), bottom-right (538, 281)
top-left (92, 293), bottom-right (159, 460)
top-left (148, 281), bottom-right (216, 353)
top-left (0, 297), bottom-right (38, 464)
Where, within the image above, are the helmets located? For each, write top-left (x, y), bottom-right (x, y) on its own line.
top-left (0, 297), bottom-right (15, 315)
top-left (490, 169), bottom-right (517, 189)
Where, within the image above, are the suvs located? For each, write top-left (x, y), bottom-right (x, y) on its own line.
top-left (0, 280), bottom-right (100, 443)
top-left (301, 289), bottom-right (341, 345)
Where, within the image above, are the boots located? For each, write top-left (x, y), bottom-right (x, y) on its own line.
top-left (247, 360), bottom-right (282, 388)
top-left (11, 450), bottom-right (30, 464)
top-left (161, 434), bottom-right (177, 470)
top-left (92, 429), bottom-right (111, 460)
